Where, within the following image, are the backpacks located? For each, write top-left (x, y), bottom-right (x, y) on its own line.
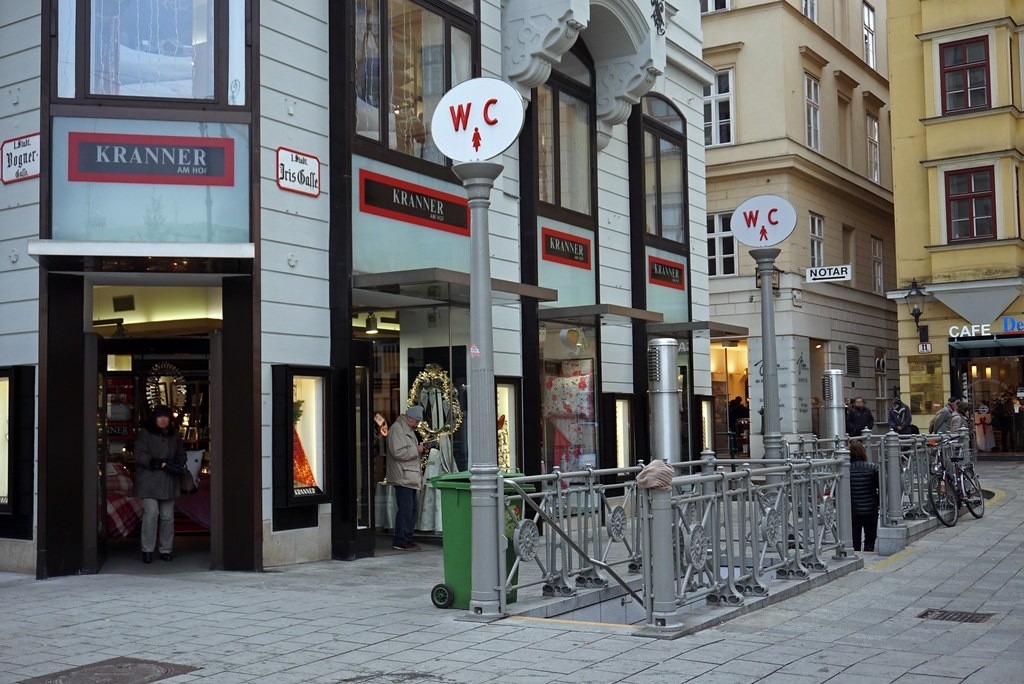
top-left (929, 409), bottom-right (949, 434)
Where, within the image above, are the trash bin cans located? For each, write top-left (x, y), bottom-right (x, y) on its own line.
top-left (426, 470), bottom-right (537, 609)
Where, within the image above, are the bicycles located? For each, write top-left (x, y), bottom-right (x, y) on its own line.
top-left (927, 436), bottom-right (985, 527)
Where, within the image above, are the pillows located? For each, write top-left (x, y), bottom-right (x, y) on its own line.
top-left (544, 375), bottom-right (594, 420)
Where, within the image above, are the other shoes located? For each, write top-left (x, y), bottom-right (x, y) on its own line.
top-left (408, 543), bottom-right (420, 549)
top-left (392, 545), bottom-right (412, 551)
top-left (159, 552), bottom-right (173, 561)
top-left (143, 553), bottom-right (153, 564)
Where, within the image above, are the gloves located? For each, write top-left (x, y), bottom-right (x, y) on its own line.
top-left (150, 458), bottom-right (163, 470)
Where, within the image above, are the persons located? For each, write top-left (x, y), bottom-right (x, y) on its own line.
top-left (849, 439), bottom-right (879, 551)
top-left (124, 405), bottom-right (188, 563)
top-left (930, 397), bottom-right (970, 466)
top-left (846, 397), bottom-right (874, 437)
top-left (386, 406), bottom-right (430, 551)
top-left (888, 397), bottom-right (912, 434)
top-left (729, 396), bottom-right (749, 451)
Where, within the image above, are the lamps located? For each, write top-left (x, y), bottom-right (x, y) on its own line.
top-left (365, 310), bottom-right (378, 335)
top-left (903, 276), bottom-right (930, 343)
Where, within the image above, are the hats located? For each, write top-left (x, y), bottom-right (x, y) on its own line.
top-left (948, 397), bottom-right (959, 404)
top-left (152, 405), bottom-right (172, 419)
top-left (406, 406), bottom-right (423, 421)
top-left (892, 397), bottom-right (901, 405)
